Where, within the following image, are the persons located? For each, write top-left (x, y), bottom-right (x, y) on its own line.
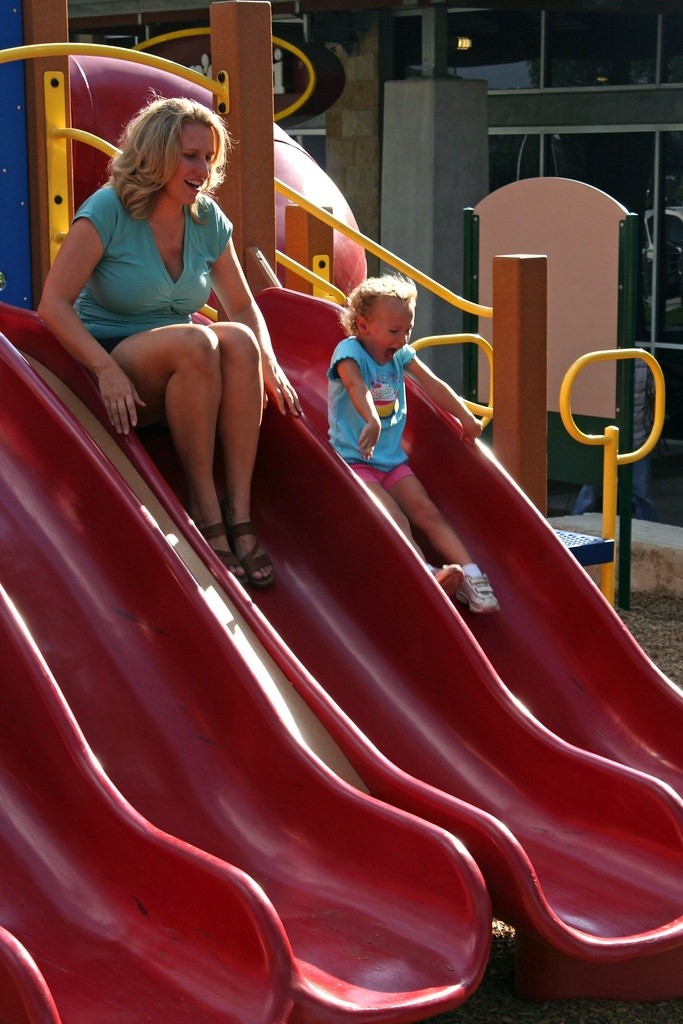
top-left (326, 276), bottom-right (500, 613)
top-left (38, 97), bottom-right (304, 587)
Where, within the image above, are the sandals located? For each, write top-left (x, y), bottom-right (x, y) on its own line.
top-left (199, 522), bottom-right (249, 584)
top-left (225, 516), bottom-right (274, 585)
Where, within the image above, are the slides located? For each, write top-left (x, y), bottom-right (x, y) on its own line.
top-left (1, 287), bottom-right (683, 1024)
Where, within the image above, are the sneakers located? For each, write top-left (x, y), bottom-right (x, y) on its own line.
top-left (456, 573), bottom-right (501, 614)
top-left (430, 563), bottom-right (464, 600)
